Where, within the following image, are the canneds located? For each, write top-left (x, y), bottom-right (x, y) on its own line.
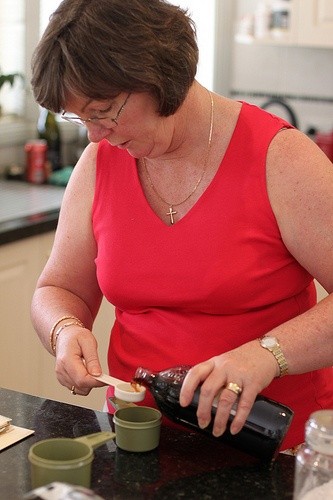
top-left (24, 139), bottom-right (48, 184)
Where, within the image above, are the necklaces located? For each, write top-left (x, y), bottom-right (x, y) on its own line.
top-left (141, 87), bottom-right (214, 224)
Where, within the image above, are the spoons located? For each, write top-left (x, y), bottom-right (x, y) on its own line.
top-left (91, 375), bottom-right (145, 402)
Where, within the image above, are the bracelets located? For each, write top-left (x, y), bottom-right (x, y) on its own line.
top-left (53, 322), bottom-right (85, 357)
top-left (49, 315), bottom-right (85, 358)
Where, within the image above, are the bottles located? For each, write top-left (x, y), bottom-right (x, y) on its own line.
top-left (132, 365), bottom-right (295, 466)
top-left (38, 108), bottom-right (61, 171)
top-left (291, 409), bottom-right (332, 500)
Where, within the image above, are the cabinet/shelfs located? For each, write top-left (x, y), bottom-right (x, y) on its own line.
top-left (1, 229), bottom-right (115, 412)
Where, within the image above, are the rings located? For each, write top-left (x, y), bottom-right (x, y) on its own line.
top-left (70, 385), bottom-right (77, 395)
top-left (225, 382), bottom-right (242, 395)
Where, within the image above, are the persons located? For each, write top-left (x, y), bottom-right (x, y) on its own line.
top-left (29, 0), bottom-right (333, 454)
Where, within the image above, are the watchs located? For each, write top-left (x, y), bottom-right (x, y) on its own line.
top-left (255, 335), bottom-right (289, 378)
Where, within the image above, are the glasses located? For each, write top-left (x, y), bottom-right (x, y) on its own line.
top-left (61, 92), bottom-right (131, 128)
